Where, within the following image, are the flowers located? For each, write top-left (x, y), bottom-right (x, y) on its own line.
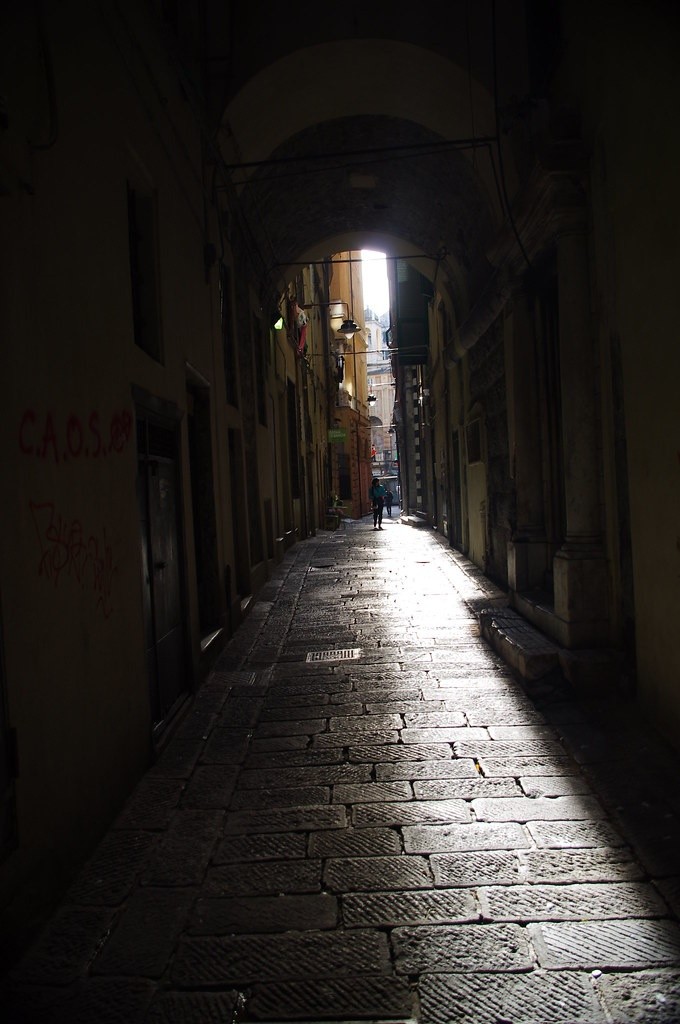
top-left (328, 508), bottom-right (343, 517)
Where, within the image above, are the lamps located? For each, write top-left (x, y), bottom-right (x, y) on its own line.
top-left (301, 302), bottom-right (361, 340)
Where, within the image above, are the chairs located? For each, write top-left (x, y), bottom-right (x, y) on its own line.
top-left (322, 503), bottom-right (337, 531)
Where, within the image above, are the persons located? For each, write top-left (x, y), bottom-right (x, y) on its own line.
top-left (369, 478), bottom-right (386, 530)
top-left (385, 489), bottom-right (393, 517)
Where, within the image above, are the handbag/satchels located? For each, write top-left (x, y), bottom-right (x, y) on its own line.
top-left (372, 500), bottom-right (378, 510)
